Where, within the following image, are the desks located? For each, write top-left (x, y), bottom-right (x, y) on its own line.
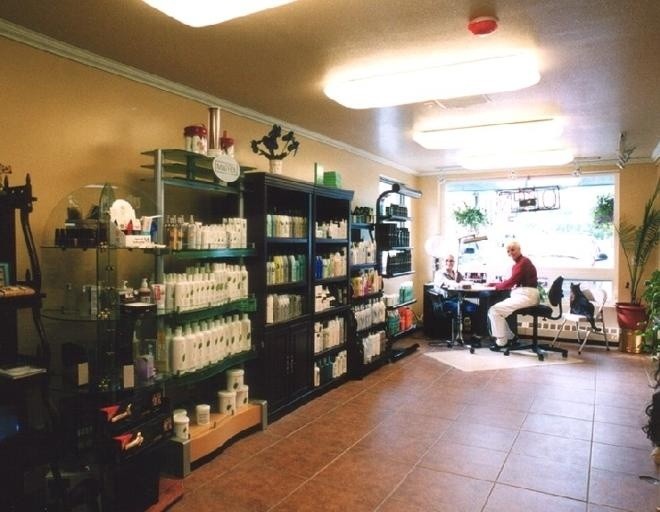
top-left (447, 286), bottom-right (511, 353)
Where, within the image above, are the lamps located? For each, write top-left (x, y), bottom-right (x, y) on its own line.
top-left (455, 233), bottom-right (488, 283)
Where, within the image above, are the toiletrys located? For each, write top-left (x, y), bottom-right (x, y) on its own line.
top-left (153, 206), bottom-right (415, 404)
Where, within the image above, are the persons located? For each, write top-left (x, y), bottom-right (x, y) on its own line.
top-left (482, 239), bottom-right (540, 351)
top-left (433, 253), bottom-right (487, 348)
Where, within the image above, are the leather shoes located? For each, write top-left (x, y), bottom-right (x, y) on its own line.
top-left (488, 334), bottom-right (521, 351)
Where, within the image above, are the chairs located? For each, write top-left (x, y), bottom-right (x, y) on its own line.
top-left (422, 284), bottom-right (446, 337)
top-left (503, 276), bottom-right (568, 361)
top-left (553, 285), bottom-right (610, 354)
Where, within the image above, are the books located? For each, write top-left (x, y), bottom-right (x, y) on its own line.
top-left (0, 261), bottom-right (10, 289)
top-left (0, 283), bottom-right (36, 298)
top-left (0, 364), bottom-right (50, 381)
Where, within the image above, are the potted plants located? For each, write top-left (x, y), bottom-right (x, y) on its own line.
top-left (250, 124), bottom-right (301, 176)
top-left (609, 176), bottom-right (660, 329)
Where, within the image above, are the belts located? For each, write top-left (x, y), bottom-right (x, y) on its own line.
top-left (514, 283), bottom-right (538, 288)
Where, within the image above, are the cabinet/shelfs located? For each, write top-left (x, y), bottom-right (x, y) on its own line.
top-left (0, 148), bottom-right (420, 512)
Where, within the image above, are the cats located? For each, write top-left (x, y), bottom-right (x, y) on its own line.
top-left (569, 282), bottom-right (601, 331)
